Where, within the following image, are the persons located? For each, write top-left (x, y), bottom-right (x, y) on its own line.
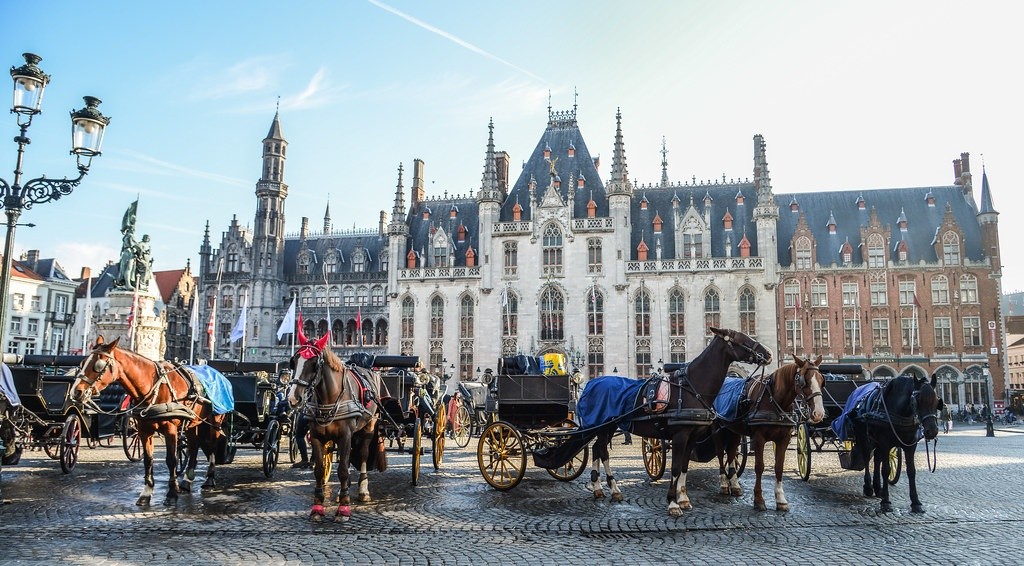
top-left (937, 394), bottom-right (1017, 434)
top-left (621, 432), bottom-right (633, 444)
top-left (447, 392), bottom-right (463, 440)
top-left (387, 351), bottom-right (439, 426)
top-left (113, 225), bottom-right (153, 290)
top-left (252, 368), bottom-right (293, 425)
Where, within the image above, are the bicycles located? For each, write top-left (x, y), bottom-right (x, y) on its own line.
top-left (933, 407), bottom-right (1018, 426)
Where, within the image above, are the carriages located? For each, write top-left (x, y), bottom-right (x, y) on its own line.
top-left (235, 359), bottom-right (578, 472)
top-left (796, 362), bottom-right (944, 515)
top-left (476, 326), bottom-right (774, 507)
top-left (0, 351), bottom-right (137, 467)
top-left (69, 334), bottom-right (283, 507)
top-left (641, 354), bottom-right (826, 514)
top-left (288, 307), bottom-right (426, 525)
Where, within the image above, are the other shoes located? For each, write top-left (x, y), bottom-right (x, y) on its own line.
top-left (944, 430), bottom-right (947, 433)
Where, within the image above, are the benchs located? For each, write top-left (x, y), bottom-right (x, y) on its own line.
top-left (99, 382), bottom-right (126, 407)
top-left (373, 356), bottom-right (420, 385)
top-left (208, 360), bottom-right (278, 389)
top-left (23, 354), bottom-right (89, 382)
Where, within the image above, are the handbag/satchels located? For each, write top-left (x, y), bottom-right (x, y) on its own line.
top-left (949, 421), bottom-right (953, 430)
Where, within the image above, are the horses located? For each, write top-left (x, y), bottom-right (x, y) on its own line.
top-left (840, 372), bottom-right (943, 514)
top-left (576, 326), bottom-right (826, 517)
top-left (285, 312), bottom-right (386, 522)
top-left (68, 335), bottom-right (234, 506)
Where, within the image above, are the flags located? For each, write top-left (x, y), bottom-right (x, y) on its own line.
top-left (794, 287), bottom-right (800, 312)
top-left (276, 293), bottom-right (296, 341)
top-left (230, 291), bottom-right (247, 344)
top-left (208, 303), bottom-right (216, 351)
top-left (120, 200), bottom-right (138, 235)
top-left (126, 288), bottom-right (139, 341)
top-left (357, 304), bottom-right (361, 331)
top-left (913, 287), bottom-right (923, 308)
top-left (327, 309), bottom-right (334, 347)
top-left (189, 285), bottom-right (199, 342)
top-left (84, 269), bottom-right (93, 336)
